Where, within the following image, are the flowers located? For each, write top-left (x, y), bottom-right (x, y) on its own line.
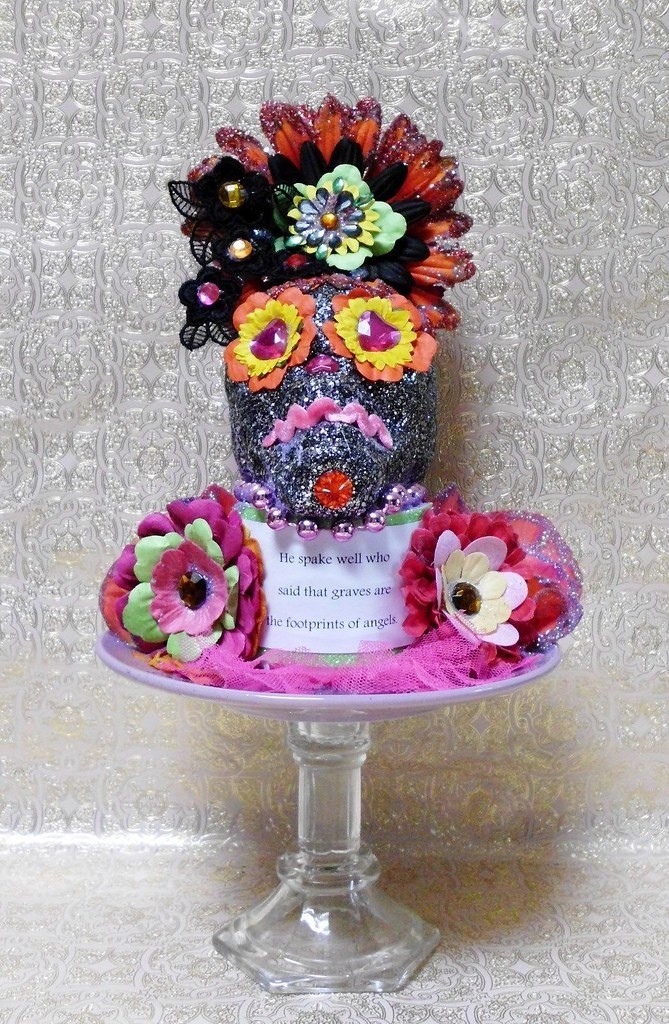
top-left (97, 96), bottom-right (566, 664)
